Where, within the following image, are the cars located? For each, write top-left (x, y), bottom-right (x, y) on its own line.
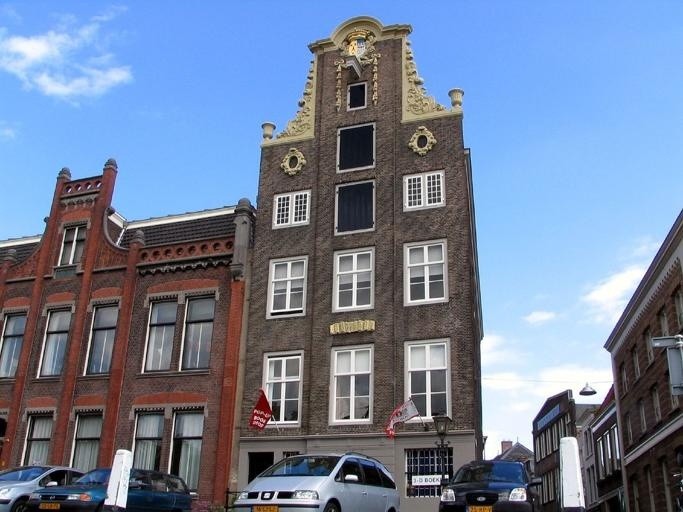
top-left (0, 465), bottom-right (84, 511)
top-left (26, 466), bottom-right (192, 511)
top-left (438, 458), bottom-right (534, 512)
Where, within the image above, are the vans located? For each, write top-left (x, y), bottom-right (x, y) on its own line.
top-left (232, 453), bottom-right (399, 512)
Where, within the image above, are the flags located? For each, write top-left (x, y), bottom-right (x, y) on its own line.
top-left (248, 389), bottom-right (273, 432)
top-left (385, 399), bottom-right (420, 440)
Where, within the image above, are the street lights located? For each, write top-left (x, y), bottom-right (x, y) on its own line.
top-left (431, 414), bottom-right (452, 495)
